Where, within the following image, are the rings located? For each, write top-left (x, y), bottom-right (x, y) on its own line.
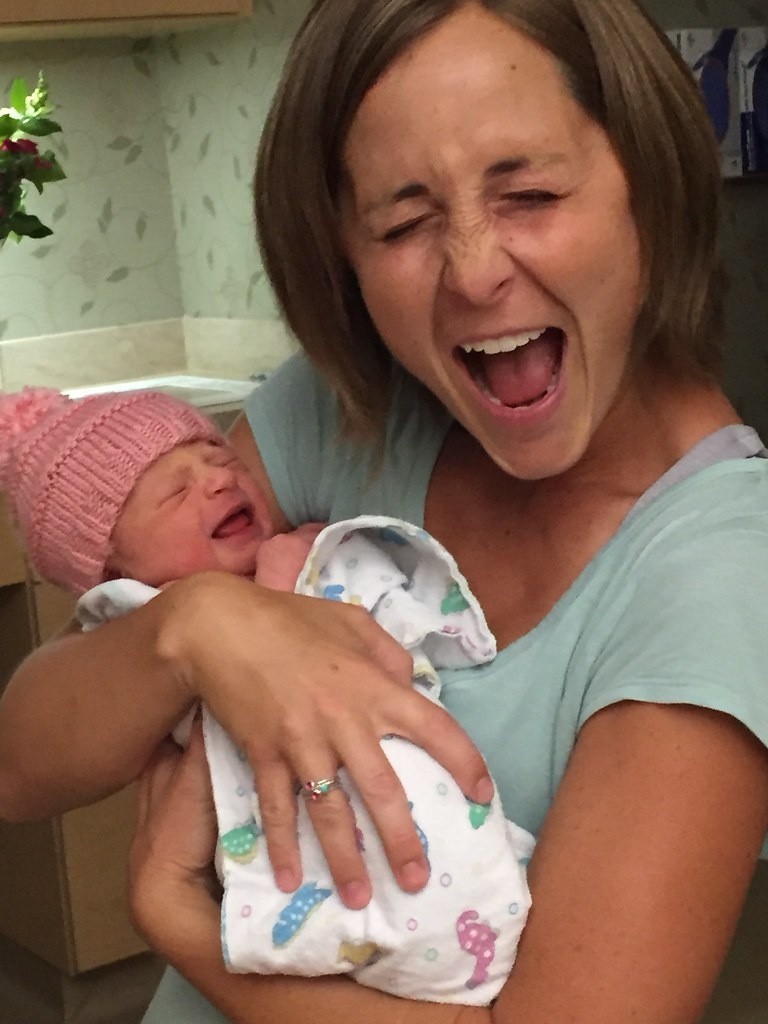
top-left (303, 776), bottom-right (340, 800)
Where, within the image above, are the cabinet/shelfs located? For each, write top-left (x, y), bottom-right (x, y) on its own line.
top-left (0, 541), bottom-right (160, 979)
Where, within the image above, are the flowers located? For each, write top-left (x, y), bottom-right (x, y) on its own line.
top-left (0, 65), bottom-right (69, 249)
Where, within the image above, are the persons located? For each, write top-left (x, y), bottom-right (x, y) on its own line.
top-left (0, 0), bottom-right (768, 1023)
top-left (0, 387), bottom-right (537, 1005)
